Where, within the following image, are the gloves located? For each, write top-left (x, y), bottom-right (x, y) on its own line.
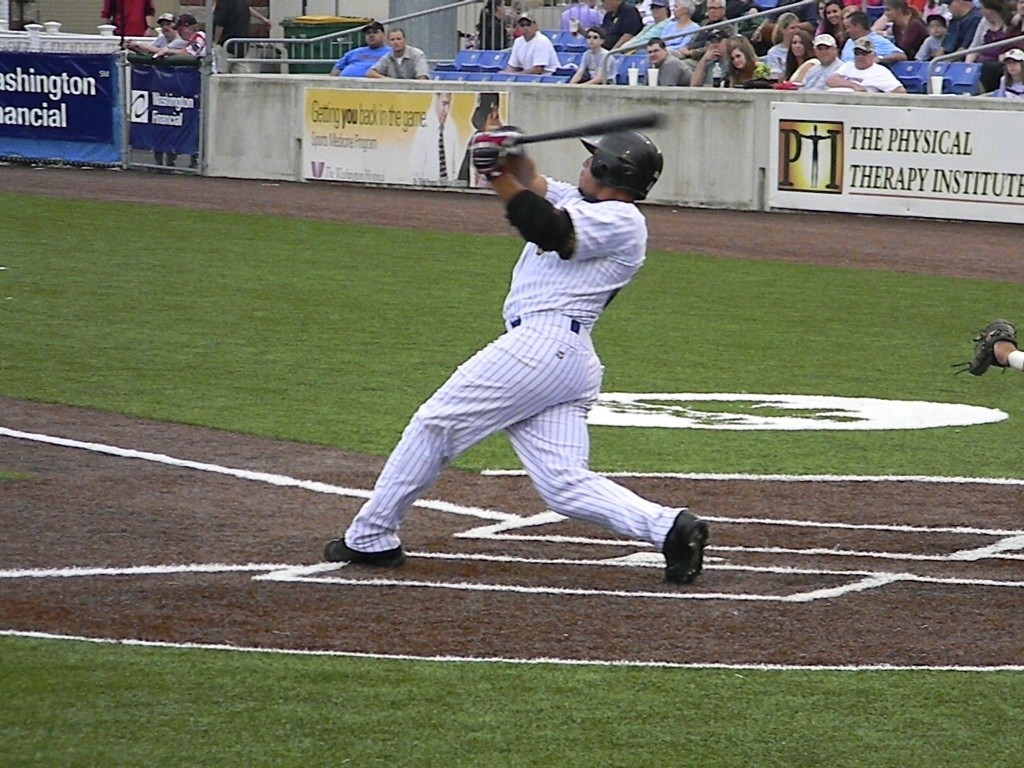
top-left (491, 124), bottom-right (524, 161)
top-left (471, 132), bottom-right (507, 180)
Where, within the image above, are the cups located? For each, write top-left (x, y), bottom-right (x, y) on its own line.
top-left (569, 19), bottom-right (579, 32)
top-left (647, 69), bottom-right (659, 86)
top-left (311, 161), bottom-right (324, 177)
top-left (931, 76), bottom-right (942, 94)
top-left (628, 68), bottom-right (639, 86)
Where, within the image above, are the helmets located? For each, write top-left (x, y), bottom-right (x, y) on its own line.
top-left (580, 127), bottom-right (663, 200)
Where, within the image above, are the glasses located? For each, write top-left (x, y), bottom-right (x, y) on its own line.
top-left (706, 6), bottom-right (723, 11)
top-left (854, 51), bottom-right (871, 56)
top-left (817, 46), bottom-right (829, 51)
top-left (520, 23), bottom-right (531, 27)
top-left (586, 35), bottom-right (601, 40)
top-left (366, 30), bottom-right (379, 34)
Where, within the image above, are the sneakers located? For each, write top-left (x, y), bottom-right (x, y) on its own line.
top-left (660, 510), bottom-right (708, 584)
top-left (324, 538), bottom-right (406, 569)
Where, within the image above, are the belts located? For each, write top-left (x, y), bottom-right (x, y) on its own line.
top-left (510, 318), bottom-right (579, 333)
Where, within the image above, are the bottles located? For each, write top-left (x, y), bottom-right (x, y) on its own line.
top-left (770, 64), bottom-right (779, 89)
top-left (712, 63), bottom-right (721, 88)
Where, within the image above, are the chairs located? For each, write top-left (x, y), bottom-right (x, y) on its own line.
top-left (888, 60), bottom-right (983, 95)
top-left (755, 0), bottom-right (779, 12)
top-left (429, 29), bottom-right (654, 85)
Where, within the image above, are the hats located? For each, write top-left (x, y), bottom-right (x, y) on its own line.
top-left (173, 15), bottom-right (197, 30)
top-left (363, 22), bottom-right (383, 32)
top-left (517, 12), bottom-right (535, 23)
top-left (813, 34), bottom-right (837, 50)
top-left (158, 13), bottom-right (175, 23)
top-left (999, 49), bottom-right (1023, 64)
top-left (926, 14), bottom-right (946, 28)
top-left (852, 39), bottom-right (875, 52)
top-left (648, 0), bottom-right (669, 8)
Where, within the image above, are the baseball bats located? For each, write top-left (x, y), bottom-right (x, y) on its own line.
top-left (512, 106), bottom-right (670, 145)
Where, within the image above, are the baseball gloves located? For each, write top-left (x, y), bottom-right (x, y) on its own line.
top-left (969, 319), bottom-right (1018, 376)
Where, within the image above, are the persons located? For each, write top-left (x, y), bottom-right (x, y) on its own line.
top-left (647, 37), bottom-right (692, 86)
top-left (693, 36), bottom-right (771, 86)
top-left (778, 30), bottom-right (821, 85)
top-left (825, 37), bottom-right (906, 92)
top-left (126, 11), bottom-right (206, 170)
top-left (330, 21), bottom-right (428, 80)
top-left (479, 0), bottom-right (507, 51)
top-left (999, 48), bottom-right (1024, 100)
top-left (800, 33), bottom-right (843, 92)
top-left (497, 12), bottom-right (562, 75)
top-left (324, 128), bottom-right (709, 585)
top-left (971, 319), bottom-right (1024, 375)
top-left (101, 1), bottom-right (155, 37)
top-left (568, 26), bottom-right (616, 85)
top-left (560, 0), bottom-right (1024, 63)
top-left (213, 0), bottom-right (271, 59)
